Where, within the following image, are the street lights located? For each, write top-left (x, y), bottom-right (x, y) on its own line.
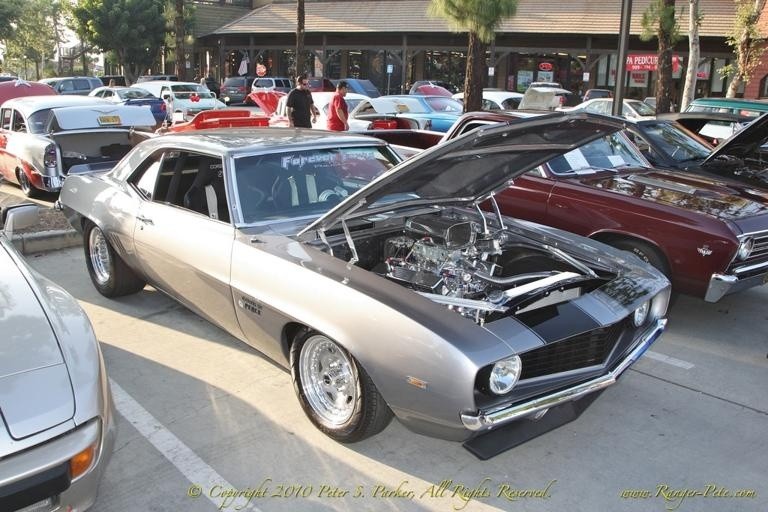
top-left (20, 53), bottom-right (30, 74)
top-left (46, 0), bottom-right (63, 72)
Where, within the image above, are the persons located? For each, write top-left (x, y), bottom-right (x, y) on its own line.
top-left (326, 79), bottom-right (350, 132)
top-left (104, 77), bottom-right (117, 97)
top-left (285, 75), bottom-right (317, 129)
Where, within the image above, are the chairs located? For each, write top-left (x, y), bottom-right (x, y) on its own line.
top-left (184, 169), bottom-right (339, 222)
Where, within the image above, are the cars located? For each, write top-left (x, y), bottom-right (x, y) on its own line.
top-left (541, 81), bottom-right (757, 126)
top-left (297, 73), bottom-right (536, 145)
top-left (58, 117), bottom-right (675, 445)
top-left (1, 228), bottom-right (111, 512)
top-left (0, 75), bottom-right (289, 186)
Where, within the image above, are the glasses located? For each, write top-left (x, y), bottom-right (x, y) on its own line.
top-left (302, 83), bottom-right (309, 86)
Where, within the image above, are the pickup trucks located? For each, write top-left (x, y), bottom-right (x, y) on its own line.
top-left (613, 108), bottom-right (767, 191)
top-left (344, 107), bottom-right (767, 294)
